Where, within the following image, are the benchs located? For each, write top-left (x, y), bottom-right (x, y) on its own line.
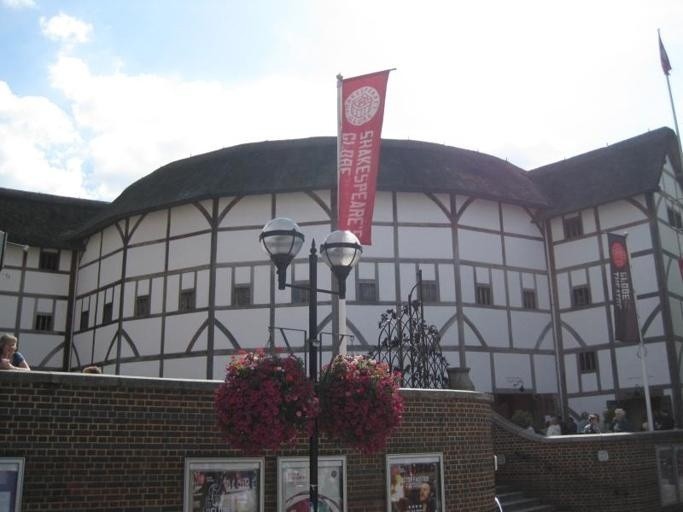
top-left (659, 36), bottom-right (672, 77)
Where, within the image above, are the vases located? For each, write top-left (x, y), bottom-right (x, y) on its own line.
top-left (211, 351), bottom-right (406, 455)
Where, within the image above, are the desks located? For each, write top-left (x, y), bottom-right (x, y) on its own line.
top-left (259, 217), bottom-right (363, 512)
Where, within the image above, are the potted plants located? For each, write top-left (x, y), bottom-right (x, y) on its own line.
top-left (0, 457), bottom-right (26, 512)
top-left (183, 455), bottom-right (348, 512)
top-left (386, 452), bottom-right (446, 512)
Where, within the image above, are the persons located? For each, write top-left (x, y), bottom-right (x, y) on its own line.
top-left (0, 334), bottom-right (30, 371)
top-left (419, 480), bottom-right (436, 512)
top-left (83, 366), bottom-right (101, 374)
top-left (527, 407), bottom-right (675, 437)
